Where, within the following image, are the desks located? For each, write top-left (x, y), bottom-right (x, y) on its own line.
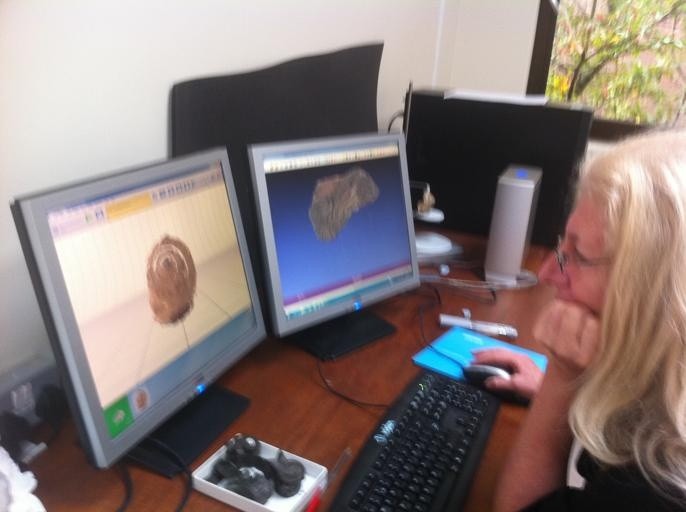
top-left (0, 225), bottom-right (554, 512)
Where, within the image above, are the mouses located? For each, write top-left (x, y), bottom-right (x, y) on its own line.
top-left (463, 365), bottom-right (530, 406)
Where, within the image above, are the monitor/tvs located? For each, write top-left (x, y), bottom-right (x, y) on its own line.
top-left (245, 132), bottom-right (421, 361)
top-left (10, 147), bottom-right (270, 479)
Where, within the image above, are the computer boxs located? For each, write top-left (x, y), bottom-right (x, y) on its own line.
top-left (402, 88), bottom-right (596, 248)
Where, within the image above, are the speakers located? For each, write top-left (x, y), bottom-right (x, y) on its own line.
top-left (480, 163), bottom-right (543, 286)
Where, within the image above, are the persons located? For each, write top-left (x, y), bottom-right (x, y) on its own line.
top-left (470, 127), bottom-right (686, 511)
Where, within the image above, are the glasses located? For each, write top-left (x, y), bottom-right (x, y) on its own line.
top-left (554, 233), bottom-right (612, 274)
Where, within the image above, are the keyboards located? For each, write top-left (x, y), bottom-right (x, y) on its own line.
top-left (329, 368), bottom-right (500, 512)
